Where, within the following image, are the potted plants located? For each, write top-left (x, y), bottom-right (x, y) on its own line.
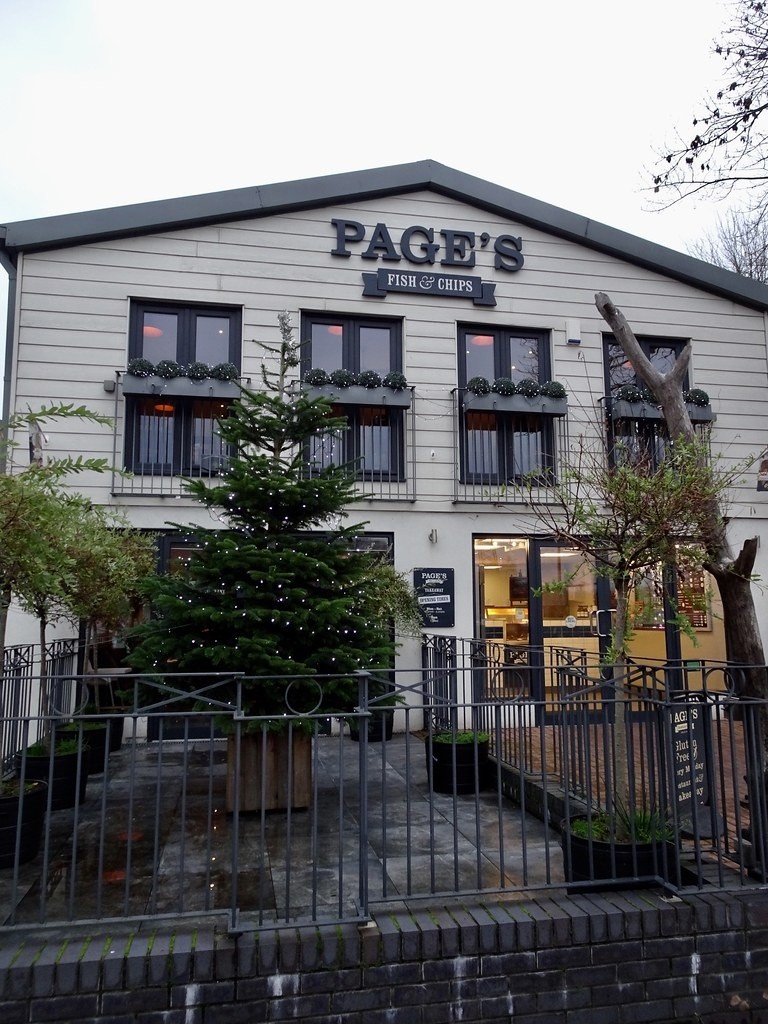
top-left (510, 349), bottom-right (767, 902)
top-left (0, 402), bottom-right (159, 868)
top-left (344, 541), bottom-right (438, 744)
top-left (118, 311), bottom-right (400, 814)
top-left (424, 731), bottom-right (490, 795)
